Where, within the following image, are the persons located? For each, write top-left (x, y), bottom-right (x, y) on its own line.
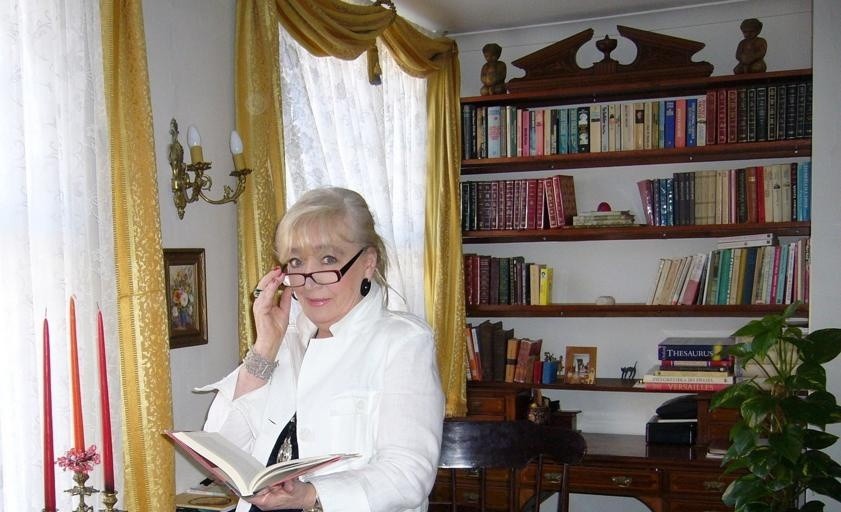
top-left (479, 43), bottom-right (507, 96)
top-left (190, 187), bottom-right (445, 512)
top-left (734, 18), bottom-right (768, 74)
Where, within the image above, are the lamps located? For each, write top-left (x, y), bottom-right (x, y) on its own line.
top-left (168, 119), bottom-right (253, 221)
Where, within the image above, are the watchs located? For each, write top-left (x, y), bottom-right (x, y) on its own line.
top-left (302, 496), bottom-right (323, 512)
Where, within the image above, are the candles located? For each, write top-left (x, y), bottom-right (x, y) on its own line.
top-left (43, 308), bottom-right (56, 511)
top-left (70, 292), bottom-right (85, 454)
top-left (96, 302), bottom-right (115, 492)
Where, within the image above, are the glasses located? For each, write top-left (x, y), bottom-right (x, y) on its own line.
top-left (282, 247), bottom-right (364, 287)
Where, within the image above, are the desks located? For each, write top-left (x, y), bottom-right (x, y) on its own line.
top-left (424, 391), bottom-right (794, 510)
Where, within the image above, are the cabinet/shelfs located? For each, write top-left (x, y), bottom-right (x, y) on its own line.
top-left (463, 67), bottom-right (809, 393)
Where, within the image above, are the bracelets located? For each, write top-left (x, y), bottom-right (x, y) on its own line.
top-left (241, 344), bottom-right (278, 381)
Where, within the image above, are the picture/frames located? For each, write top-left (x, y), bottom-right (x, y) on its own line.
top-left (161, 247), bottom-right (209, 351)
top-left (564, 346), bottom-right (597, 385)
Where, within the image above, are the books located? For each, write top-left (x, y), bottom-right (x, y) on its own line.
top-left (636, 161), bottom-right (811, 226)
top-left (461, 82), bottom-right (814, 161)
top-left (458, 174), bottom-right (576, 232)
top-left (573, 210), bottom-right (636, 226)
top-left (641, 332), bottom-right (802, 391)
top-left (165, 428), bottom-right (362, 499)
top-left (463, 253), bottom-right (554, 307)
top-left (647, 234), bottom-right (810, 305)
top-left (175, 491), bottom-right (240, 511)
top-left (464, 320), bottom-right (559, 385)
top-left (705, 438), bottom-right (749, 460)
